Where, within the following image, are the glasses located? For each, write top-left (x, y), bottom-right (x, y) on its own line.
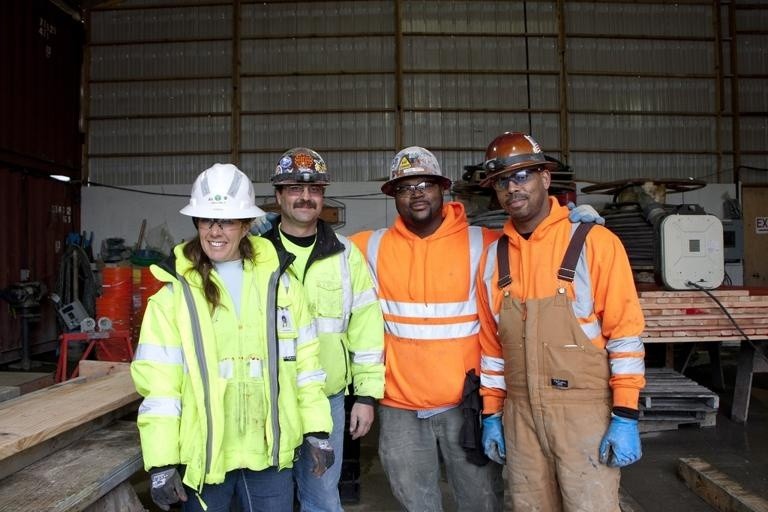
top-left (490, 168), bottom-right (535, 190)
top-left (195, 217), bottom-right (244, 234)
top-left (395, 181), bottom-right (437, 196)
top-left (289, 183), bottom-right (328, 198)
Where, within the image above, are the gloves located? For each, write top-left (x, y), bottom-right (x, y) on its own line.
top-left (483, 415), bottom-right (509, 465)
top-left (305, 433), bottom-right (335, 476)
top-left (568, 201), bottom-right (608, 226)
top-left (599, 416), bottom-right (646, 470)
top-left (149, 466), bottom-right (188, 509)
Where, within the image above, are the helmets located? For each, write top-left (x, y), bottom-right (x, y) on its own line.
top-left (272, 147), bottom-right (332, 185)
top-left (484, 131), bottom-right (558, 180)
top-left (179, 163), bottom-right (268, 221)
top-left (380, 147), bottom-right (452, 192)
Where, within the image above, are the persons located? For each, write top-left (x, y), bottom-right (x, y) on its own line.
top-left (473, 131), bottom-right (647, 511)
top-left (250, 143), bottom-right (606, 511)
top-left (127, 162), bottom-right (337, 512)
top-left (252, 146), bottom-right (388, 511)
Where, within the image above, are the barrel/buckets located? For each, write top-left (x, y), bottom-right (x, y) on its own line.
top-left (93, 250), bottom-right (164, 362)
